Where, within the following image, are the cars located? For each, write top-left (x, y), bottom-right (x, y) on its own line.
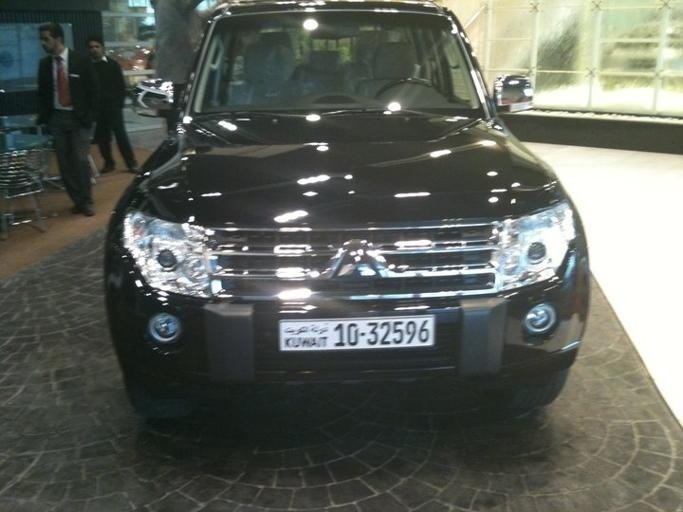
top-left (104, 1), bottom-right (588, 420)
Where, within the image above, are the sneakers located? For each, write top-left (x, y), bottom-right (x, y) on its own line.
top-left (101, 164), bottom-right (114, 173)
top-left (129, 165), bottom-right (138, 173)
top-left (72, 204), bottom-right (95, 217)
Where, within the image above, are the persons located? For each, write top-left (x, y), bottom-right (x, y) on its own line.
top-left (81, 38), bottom-right (143, 175)
top-left (34, 29), bottom-right (102, 216)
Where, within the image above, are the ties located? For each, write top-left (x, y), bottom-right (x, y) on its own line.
top-left (54, 55), bottom-right (71, 108)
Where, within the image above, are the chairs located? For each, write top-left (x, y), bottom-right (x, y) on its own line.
top-left (223, 27), bottom-right (461, 115)
